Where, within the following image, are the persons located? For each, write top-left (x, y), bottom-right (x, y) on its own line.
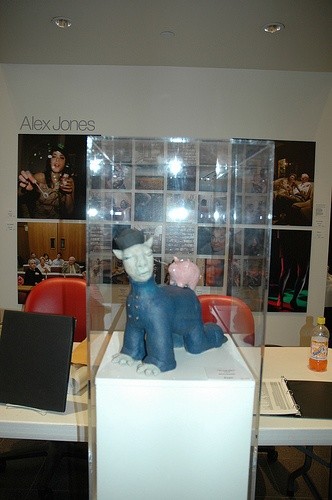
top-left (273, 174), bottom-right (312, 221)
top-left (91, 142), bottom-right (266, 287)
top-left (24, 252), bottom-right (81, 286)
top-left (19, 144), bottom-right (74, 219)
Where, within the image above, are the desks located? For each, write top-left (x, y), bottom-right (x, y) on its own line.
top-left (0, 341), bottom-right (332, 500)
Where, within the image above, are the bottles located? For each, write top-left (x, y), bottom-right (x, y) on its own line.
top-left (59, 162), bottom-right (71, 197)
top-left (308, 317), bottom-right (328, 371)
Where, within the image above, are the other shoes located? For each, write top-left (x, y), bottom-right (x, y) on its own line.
top-left (275, 299), bottom-right (283, 309)
top-left (289, 299), bottom-right (298, 311)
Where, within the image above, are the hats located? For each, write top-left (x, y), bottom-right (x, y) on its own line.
top-left (46, 143), bottom-right (64, 158)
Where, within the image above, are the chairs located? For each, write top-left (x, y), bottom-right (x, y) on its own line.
top-left (0, 277), bottom-right (107, 500)
top-left (196, 294), bottom-right (278, 460)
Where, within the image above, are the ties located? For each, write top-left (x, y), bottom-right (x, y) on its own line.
top-left (71, 265), bottom-right (74, 273)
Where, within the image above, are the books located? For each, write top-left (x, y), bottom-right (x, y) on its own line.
top-left (253, 378), bottom-right (332, 419)
